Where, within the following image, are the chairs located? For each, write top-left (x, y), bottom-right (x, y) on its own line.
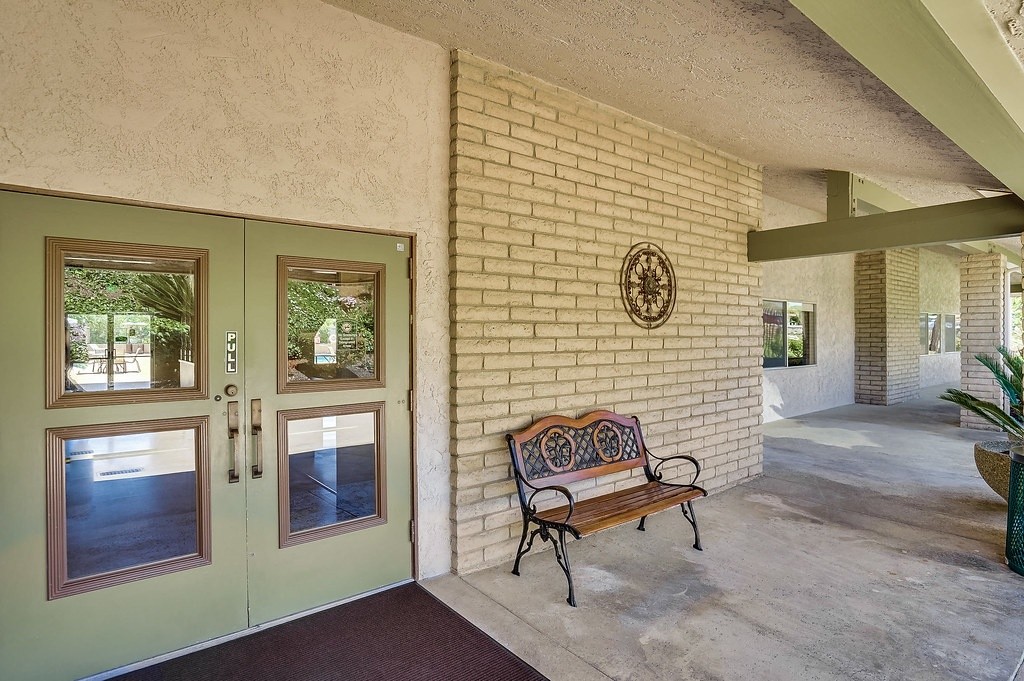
top-left (92, 347), bottom-right (141, 373)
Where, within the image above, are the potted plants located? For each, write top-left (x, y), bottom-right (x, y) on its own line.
top-left (936, 344), bottom-right (1024, 502)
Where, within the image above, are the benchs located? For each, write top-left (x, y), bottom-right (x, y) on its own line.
top-left (505, 410), bottom-right (708, 608)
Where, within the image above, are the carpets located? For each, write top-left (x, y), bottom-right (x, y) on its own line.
top-left (102, 581), bottom-right (549, 681)
